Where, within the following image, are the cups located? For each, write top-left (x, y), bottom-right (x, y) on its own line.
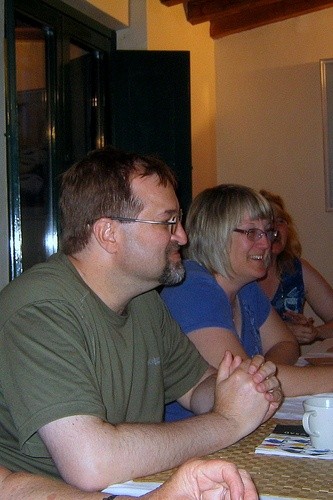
top-left (302, 396), bottom-right (333, 452)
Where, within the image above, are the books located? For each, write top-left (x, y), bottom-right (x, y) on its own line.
top-left (255, 424), bottom-right (332, 459)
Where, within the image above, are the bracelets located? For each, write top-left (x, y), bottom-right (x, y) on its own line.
top-left (102, 493), bottom-right (118, 500)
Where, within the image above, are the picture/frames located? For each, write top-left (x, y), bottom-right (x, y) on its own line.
top-left (319, 58), bottom-right (333, 213)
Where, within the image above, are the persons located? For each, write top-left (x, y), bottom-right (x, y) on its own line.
top-left (0, 457), bottom-right (260, 500)
top-left (0, 145), bottom-right (284, 494)
top-left (163, 184), bottom-right (333, 424)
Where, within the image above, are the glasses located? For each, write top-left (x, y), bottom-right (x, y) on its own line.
top-left (106, 208), bottom-right (184, 236)
top-left (232, 228), bottom-right (280, 241)
